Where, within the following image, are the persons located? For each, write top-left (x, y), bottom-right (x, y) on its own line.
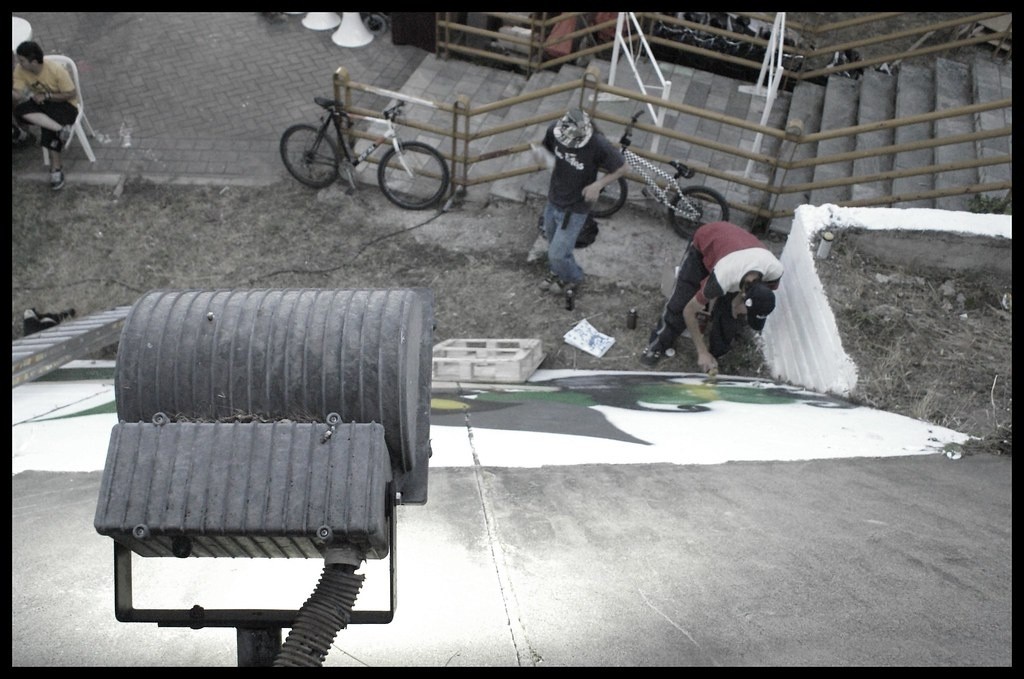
top-left (639, 222), bottom-right (783, 376)
top-left (13, 42), bottom-right (79, 191)
top-left (540, 107), bottom-right (629, 294)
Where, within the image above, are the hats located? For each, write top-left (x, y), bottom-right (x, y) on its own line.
top-left (745, 284), bottom-right (775, 331)
top-left (553, 108), bottom-right (594, 150)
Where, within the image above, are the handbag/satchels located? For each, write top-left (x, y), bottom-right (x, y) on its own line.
top-left (537, 213), bottom-right (599, 249)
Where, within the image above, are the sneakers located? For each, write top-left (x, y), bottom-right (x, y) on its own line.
top-left (549, 278), bottom-right (578, 294)
top-left (638, 336), bottom-right (663, 368)
top-left (718, 349), bottom-right (740, 375)
top-left (537, 270), bottom-right (561, 291)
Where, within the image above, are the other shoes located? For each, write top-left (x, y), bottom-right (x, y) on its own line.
top-left (49, 165), bottom-right (65, 191)
top-left (56, 123), bottom-right (75, 149)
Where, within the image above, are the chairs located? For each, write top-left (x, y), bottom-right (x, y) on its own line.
top-left (14, 54), bottom-right (98, 164)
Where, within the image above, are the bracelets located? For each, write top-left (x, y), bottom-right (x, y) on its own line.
top-left (45, 92), bottom-right (51, 100)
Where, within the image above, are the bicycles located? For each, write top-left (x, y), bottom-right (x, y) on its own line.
top-left (279, 95), bottom-right (450, 210)
top-left (654, 12), bottom-right (898, 96)
top-left (587, 109), bottom-right (732, 243)
